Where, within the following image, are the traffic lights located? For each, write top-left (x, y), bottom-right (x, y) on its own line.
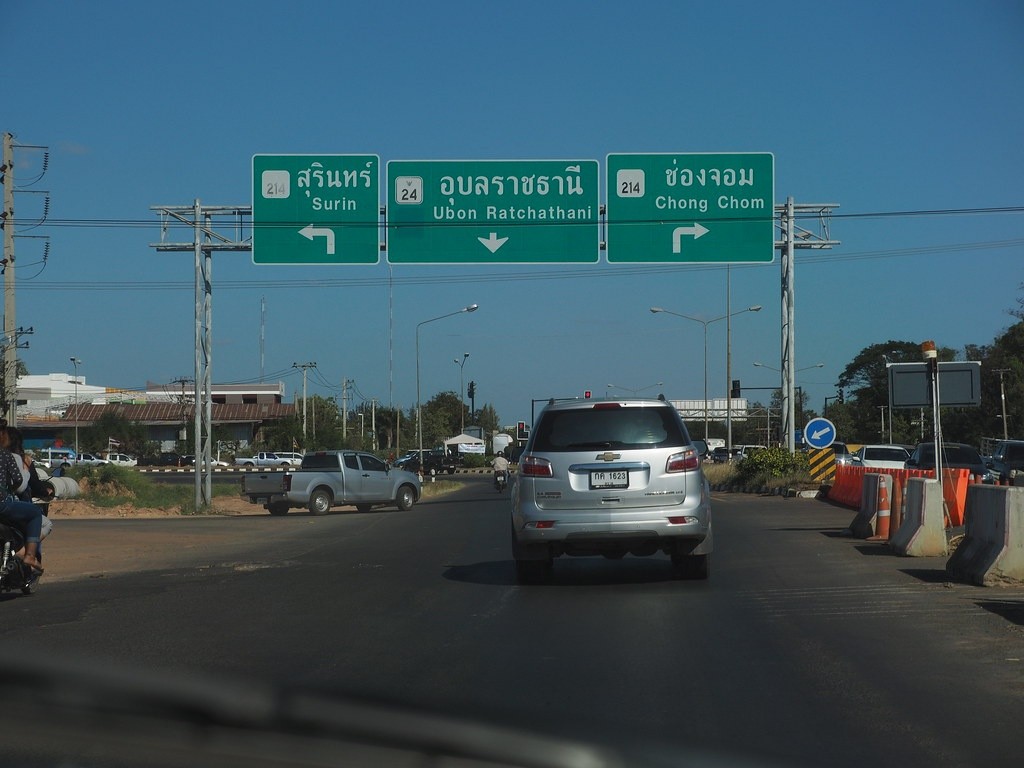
top-left (516, 420), bottom-right (530, 440)
top-left (583, 390), bottom-right (592, 399)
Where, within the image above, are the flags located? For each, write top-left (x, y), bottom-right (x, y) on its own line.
top-left (109, 438), bottom-right (120, 447)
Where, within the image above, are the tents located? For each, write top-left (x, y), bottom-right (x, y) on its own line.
top-left (443, 434), bottom-right (485, 457)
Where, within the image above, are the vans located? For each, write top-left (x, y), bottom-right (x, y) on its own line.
top-left (273, 452), bottom-right (304, 469)
top-left (32, 448), bottom-right (77, 470)
top-left (393, 450), bottom-right (457, 477)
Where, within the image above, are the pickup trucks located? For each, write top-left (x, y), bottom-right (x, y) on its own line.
top-left (980, 438), bottom-right (1024, 487)
top-left (234, 451), bottom-right (293, 469)
top-left (242, 449), bottom-right (421, 517)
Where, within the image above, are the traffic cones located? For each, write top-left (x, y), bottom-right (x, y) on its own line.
top-left (865, 476), bottom-right (890, 541)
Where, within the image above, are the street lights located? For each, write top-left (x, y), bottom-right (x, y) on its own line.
top-left (69, 356), bottom-right (82, 462)
top-left (877, 406), bottom-right (888, 444)
top-left (453, 353), bottom-right (470, 434)
top-left (650, 304), bottom-right (763, 458)
top-left (414, 303), bottom-right (479, 493)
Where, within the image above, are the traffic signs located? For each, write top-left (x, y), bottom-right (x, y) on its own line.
top-left (605, 151), bottom-right (775, 266)
top-left (250, 153), bottom-right (381, 266)
top-left (385, 158), bottom-right (600, 265)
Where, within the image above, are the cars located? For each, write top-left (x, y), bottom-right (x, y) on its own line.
top-left (850, 444), bottom-right (916, 469)
top-left (703, 437), bottom-right (768, 464)
top-left (106, 453), bottom-right (138, 468)
top-left (827, 441), bottom-right (854, 466)
top-left (904, 441), bottom-right (995, 486)
top-left (178, 454), bottom-right (229, 466)
top-left (74, 452), bottom-right (109, 468)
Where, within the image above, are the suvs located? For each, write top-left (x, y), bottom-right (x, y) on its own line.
top-left (506, 392), bottom-right (713, 588)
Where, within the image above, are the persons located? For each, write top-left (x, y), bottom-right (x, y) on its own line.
top-left (491, 451), bottom-right (509, 484)
top-left (0, 418), bottom-right (63, 571)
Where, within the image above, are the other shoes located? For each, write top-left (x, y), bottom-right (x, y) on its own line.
top-left (23, 558), bottom-right (42, 569)
top-left (16, 552), bottom-right (24, 561)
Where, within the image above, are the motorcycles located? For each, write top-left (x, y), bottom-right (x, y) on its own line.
top-left (490, 461), bottom-right (511, 494)
top-left (0, 464), bottom-right (66, 598)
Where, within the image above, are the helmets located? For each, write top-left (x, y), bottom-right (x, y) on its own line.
top-left (497, 451), bottom-right (504, 457)
